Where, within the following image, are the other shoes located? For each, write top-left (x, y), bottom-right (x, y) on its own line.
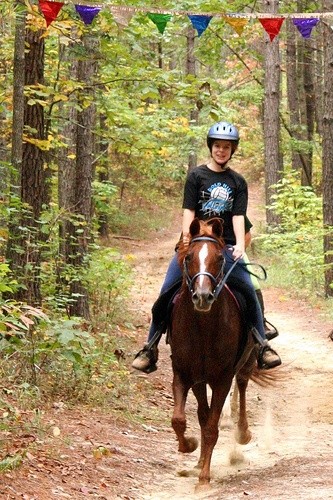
top-left (132, 346), bottom-right (160, 370)
top-left (255, 338), bottom-right (280, 366)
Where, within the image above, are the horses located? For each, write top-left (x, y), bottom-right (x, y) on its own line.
top-left (166, 216), bottom-right (296, 495)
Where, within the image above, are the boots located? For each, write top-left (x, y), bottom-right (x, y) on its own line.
top-left (255, 288), bottom-right (271, 337)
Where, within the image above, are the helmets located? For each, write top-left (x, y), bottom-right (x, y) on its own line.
top-left (207, 122), bottom-right (240, 155)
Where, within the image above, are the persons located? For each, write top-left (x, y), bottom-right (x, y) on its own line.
top-left (129, 122), bottom-right (283, 372)
top-left (244, 216), bottom-right (273, 337)
